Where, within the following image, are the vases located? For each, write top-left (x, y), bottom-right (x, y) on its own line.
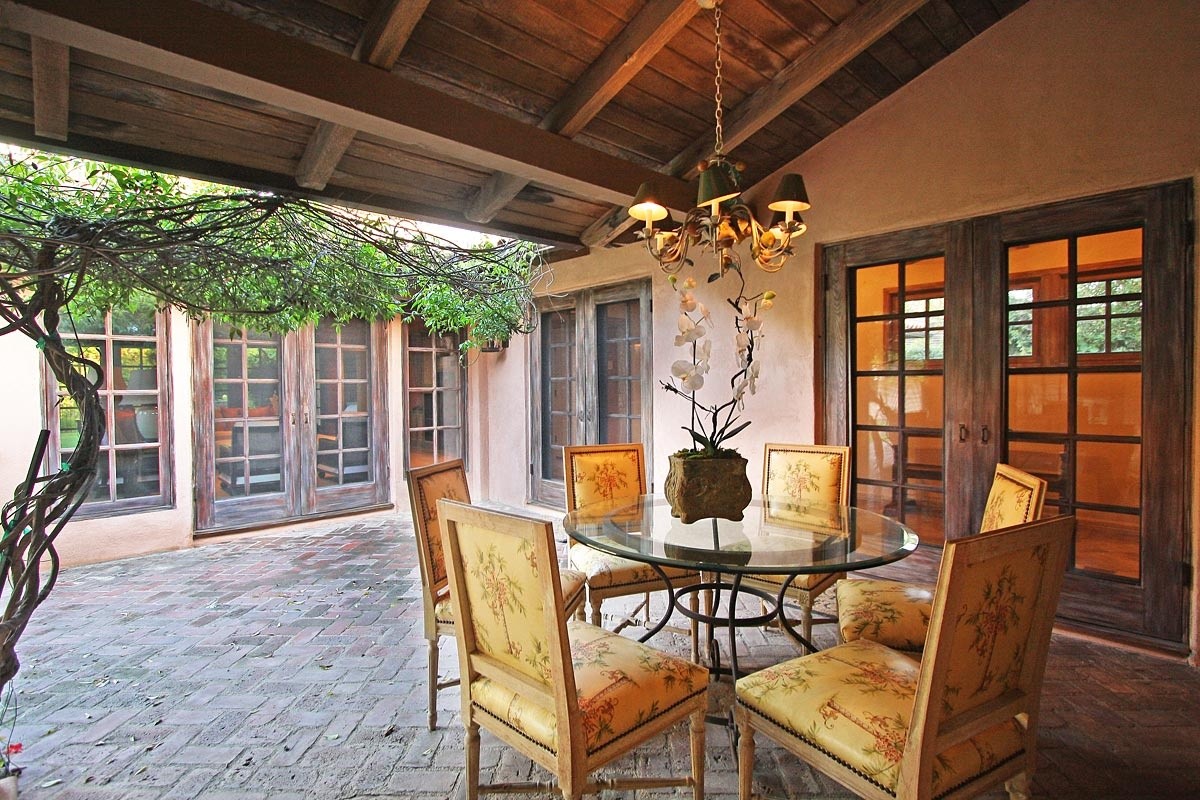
top-left (663, 449), bottom-right (754, 524)
top-left (0, 776), bottom-right (19, 800)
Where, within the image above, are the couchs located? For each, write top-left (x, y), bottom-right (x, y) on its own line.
top-left (214, 406), bottom-right (277, 440)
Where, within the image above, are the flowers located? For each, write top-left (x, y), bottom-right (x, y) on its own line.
top-left (0, 678), bottom-right (22, 779)
top-left (658, 258), bottom-right (777, 459)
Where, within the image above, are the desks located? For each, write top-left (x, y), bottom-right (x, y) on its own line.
top-left (559, 495), bottom-right (919, 780)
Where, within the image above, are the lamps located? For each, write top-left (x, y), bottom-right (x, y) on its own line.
top-left (628, 0), bottom-right (810, 282)
top-left (481, 337), bottom-right (509, 353)
top-left (118, 368), bottom-right (161, 440)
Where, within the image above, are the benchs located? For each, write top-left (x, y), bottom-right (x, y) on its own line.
top-left (883, 444), bottom-right (1068, 518)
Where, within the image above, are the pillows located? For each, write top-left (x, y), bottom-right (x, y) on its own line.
top-left (255, 405), bottom-right (274, 417)
top-left (239, 408), bottom-right (256, 418)
top-left (221, 407), bottom-right (240, 429)
top-left (215, 408), bottom-right (228, 431)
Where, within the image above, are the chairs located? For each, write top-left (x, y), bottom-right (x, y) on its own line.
top-left (403, 459), bottom-right (589, 732)
top-left (217, 423), bottom-right (283, 496)
top-left (116, 405), bottom-right (159, 497)
top-left (317, 418), bottom-right (368, 483)
top-left (435, 495), bottom-right (711, 800)
top-left (703, 442), bottom-right (852, 661)
top-left (562, 442), bottom-right (702, 666)
top-left (836, 463), bottom-right (1047, 648)
top-left (735, 512), bottom-right (1077, 800)
